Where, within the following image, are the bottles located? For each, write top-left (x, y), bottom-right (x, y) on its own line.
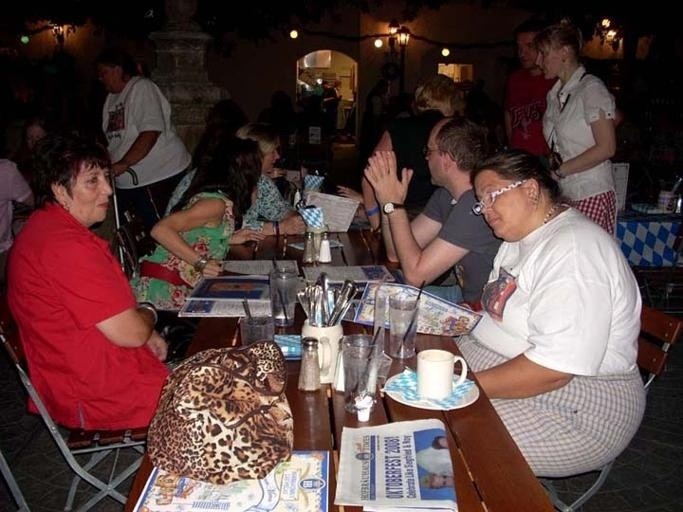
top-left (295, 336), bottom-right (322, 392)
top-left (318, 230), bottom-right (333, 264)
top-left (300, 231), bottom-right (317, 263)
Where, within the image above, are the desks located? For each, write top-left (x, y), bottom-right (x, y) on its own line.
top-left (341, 107), bottom-right (352, 130)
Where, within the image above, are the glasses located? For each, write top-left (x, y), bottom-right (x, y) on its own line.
top-left (443, 475), bottom-right (446, 485)
top-left (421, 147), bottom-right (457, 163)
top-left (472, 180), bottom-right (529, 216)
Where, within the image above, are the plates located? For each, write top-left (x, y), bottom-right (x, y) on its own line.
top-left (385, 370), bottom-right (483, 412)
top-left (268, 332), bottom-right (304, 361)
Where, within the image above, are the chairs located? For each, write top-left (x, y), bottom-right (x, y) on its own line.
top-left (0, 309), bottom-right (149, 512)
top-left (124, 187), bottom-right (161, 242)
top-left (121, 216), bottom-right (153, 277)
top-left (536, 304), bottom-right (683, 512)
top-left (632, 226), bottom-right (682, 313)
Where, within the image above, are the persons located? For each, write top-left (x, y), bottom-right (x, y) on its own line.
top-left (420, 472), bottom-right (455, 490)
top-left (2, 47), bottom-right (342, 433)
top-left (337, 14), bottom-right (648, 477)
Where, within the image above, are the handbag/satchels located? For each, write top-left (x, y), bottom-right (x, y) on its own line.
top-left (147, 339), bottom-right (294, 484)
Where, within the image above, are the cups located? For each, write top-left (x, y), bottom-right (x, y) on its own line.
top-left (341, 333), bottom-right (383, 414)
top-left (300, 318), bottom-right (341, 386)
top-left (268, 268), bottom-right (297, 326)
top-left (388, 292), bottom-right (417, 360)
top-left (238, 311), bottom-right (274, 345)
top-left (414, 347), bottom-right (467, 402)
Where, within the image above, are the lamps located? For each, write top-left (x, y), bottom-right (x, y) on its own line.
top-left (398, 27), bottom-right (412, 79)
top-left (388, 18), bottom-right (399, 59)
